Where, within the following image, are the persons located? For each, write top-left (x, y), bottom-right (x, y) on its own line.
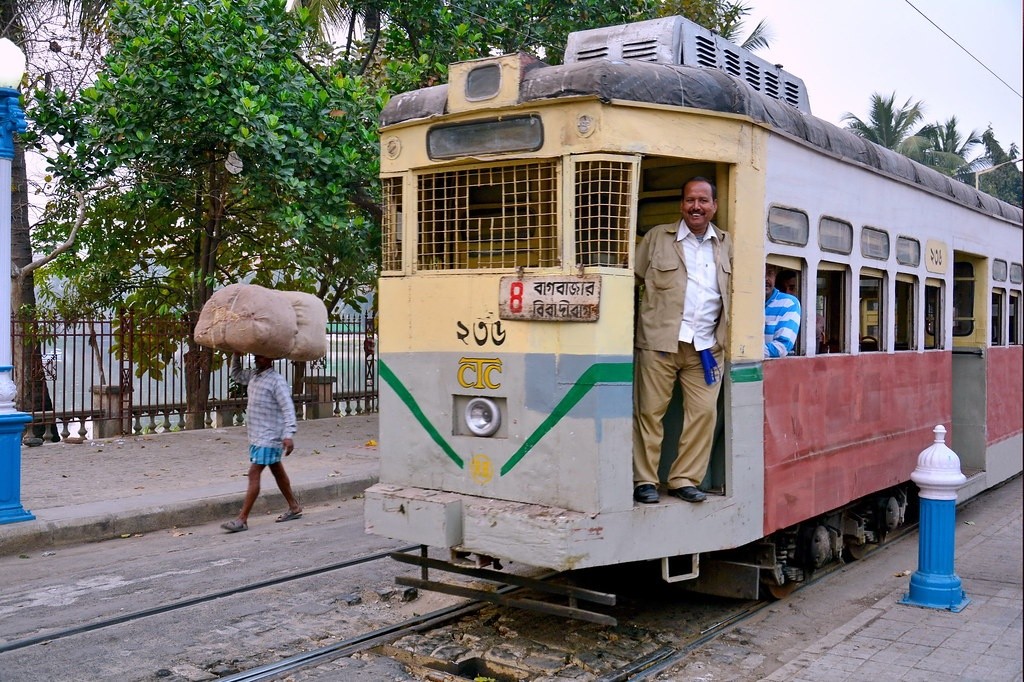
top-left (765, 265), bottom-right (825, 359)
top-left (633, 179), bottom-right (733, 504)
top-left (220, 351), bottom-right (302, 532)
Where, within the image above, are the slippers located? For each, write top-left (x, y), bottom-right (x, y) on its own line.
top-left (220, 518), bottom-right (248, 531)
top-left (275, 510), bottom-right (302, 522)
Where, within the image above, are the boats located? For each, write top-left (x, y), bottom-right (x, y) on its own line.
top-left (41, 348), bottom-right (63, 366)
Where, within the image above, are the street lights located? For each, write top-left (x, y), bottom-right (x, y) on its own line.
top-left (0, 38), bottom-right (37, 523)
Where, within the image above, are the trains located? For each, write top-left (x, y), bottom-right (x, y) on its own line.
top-left (364, 13), bottom-right (1024, 626)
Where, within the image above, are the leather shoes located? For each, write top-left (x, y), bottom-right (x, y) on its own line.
top-left (633, 485), bottom-right (658, 502)
top-left (668, 487), bottom-right (707, 501)
